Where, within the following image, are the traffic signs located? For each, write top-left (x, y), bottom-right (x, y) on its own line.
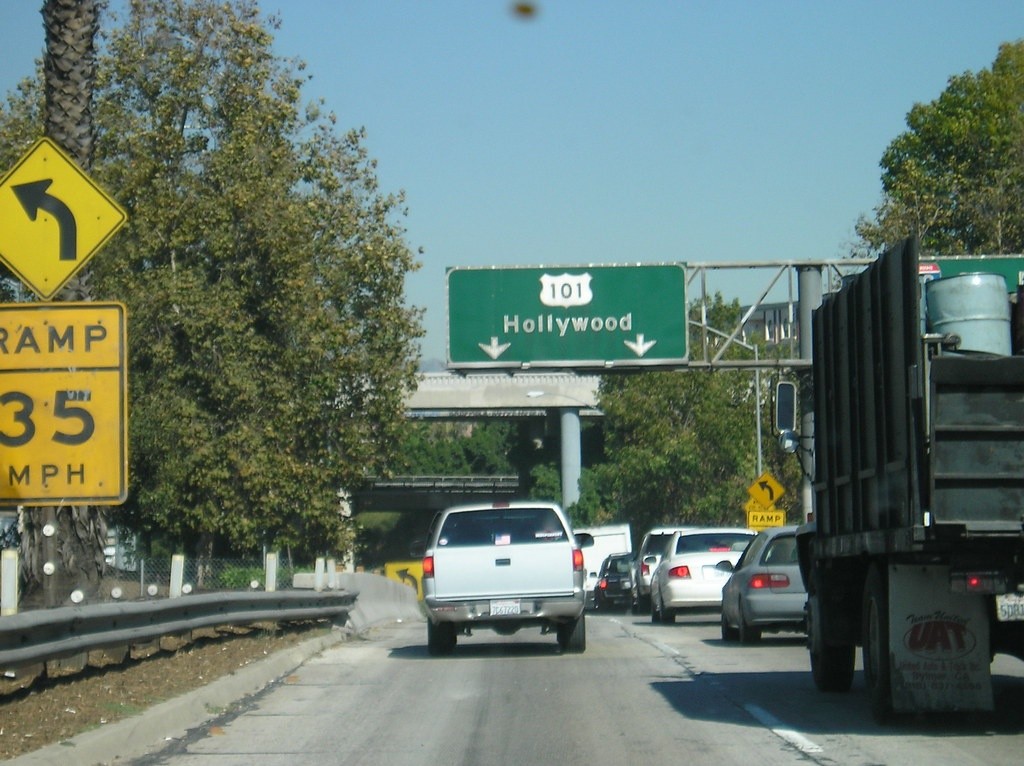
top-left (443, 268), bottom-right (695, 366)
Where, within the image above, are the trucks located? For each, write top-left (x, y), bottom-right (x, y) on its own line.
top-left (777, 233), bottom-right (1023, 720)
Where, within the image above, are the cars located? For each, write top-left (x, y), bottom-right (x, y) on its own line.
top-left (650, 528), bottom-right (761, 620)
top-left (714, 527), bottom-right (814, 644)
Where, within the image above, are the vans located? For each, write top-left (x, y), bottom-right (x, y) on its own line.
top-left (498, 524), bottom-right (632, 610)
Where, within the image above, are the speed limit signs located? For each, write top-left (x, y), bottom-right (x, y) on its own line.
top-left (0, 304), bottom-right (130, 506)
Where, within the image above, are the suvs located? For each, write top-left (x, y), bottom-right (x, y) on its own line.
top-left (589, 551), bottom-right (638, 611)
top-left (635, 526), bottom-right (696, 617)
top-left (419, 501), bottom-right (595, 654)
